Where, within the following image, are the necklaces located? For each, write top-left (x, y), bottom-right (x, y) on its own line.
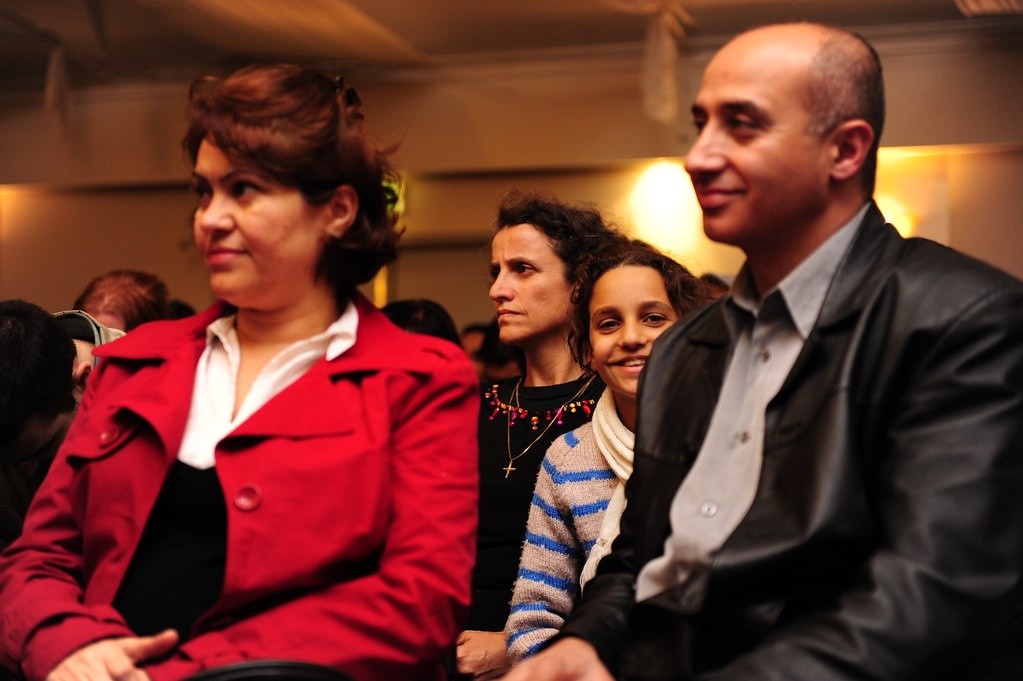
top-left (504, 374), bottom-right (597, 478)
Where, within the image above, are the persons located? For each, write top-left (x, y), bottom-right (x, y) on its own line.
top-left (502, 23), bottom-right (1023, 681)
top-left (382, 191), bottom-right (727, 681)
top-left (0, 62), bottom-right (481, 681)
top-left (0, 270), bottom-right (197, 555)
